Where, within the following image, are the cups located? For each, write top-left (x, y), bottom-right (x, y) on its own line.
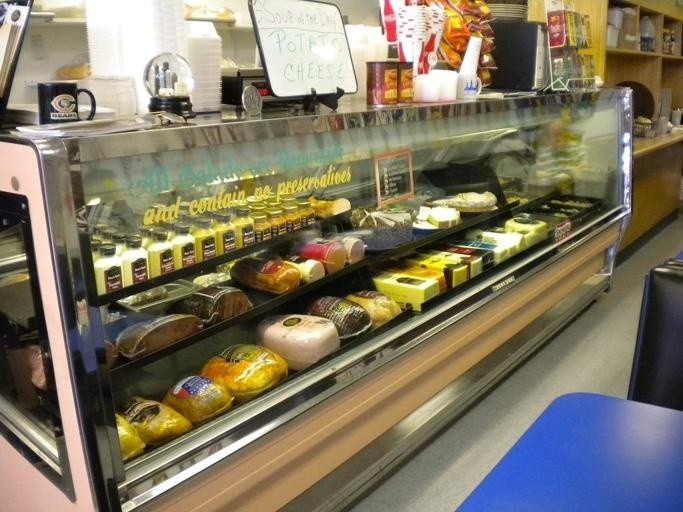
top-left (393, 4), bottom-right (446, 76)
top-left (457, 74), bottom-right (482, 99)
top-left (38, 80), bottom-right (96, 124)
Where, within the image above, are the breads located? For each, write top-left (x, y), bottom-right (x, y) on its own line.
top-left (199, 342), bottom-right (287, 403)
top-left (230, 258), bottom-right (301, 296)
top-left (104, 313), bottom-right (204, 361)
top-left (344, 291), bottom-right (402, 331)
top-left (254, 314), bottom-right (340, 371)
top-left (111, 414), bottom-right (145, 463)
top-left (338, 206), bottom-right (416, 248)
top-left (427, 207), bottom-right (461, 230)
top-left (161, 374), bottom-right (234, 426)
top-left (166, 284), bottom-right (253, 327)
top-left (119, 393), bottom-right (193, 449)
top-left (448, 192), bottom-right (498, 213)
top-left (301, 297), bottom-right (371, 345)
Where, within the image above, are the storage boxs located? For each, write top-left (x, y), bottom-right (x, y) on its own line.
top-left (607, 7), bottom-right (624, 29)
top-left (606, 25), bottom-right (619, 48)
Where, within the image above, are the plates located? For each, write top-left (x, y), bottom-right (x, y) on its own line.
top-left (413, 220), bottom-right (437, 231)
top-left (488, 3), bottom-right (528, 19)
top-left (340, 320), bottom-right (372, 339)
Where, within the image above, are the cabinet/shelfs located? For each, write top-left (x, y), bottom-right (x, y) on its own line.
top-left (527, 0), bottom-right (683, 253)
top-left (0, 86), bottom-right (633, 512)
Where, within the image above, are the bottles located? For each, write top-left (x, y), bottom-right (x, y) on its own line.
top-left (90, 190), bottom-right (317, 297)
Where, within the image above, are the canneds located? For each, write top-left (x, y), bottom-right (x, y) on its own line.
top-left (228, 191), bottom-right (316, 244)
